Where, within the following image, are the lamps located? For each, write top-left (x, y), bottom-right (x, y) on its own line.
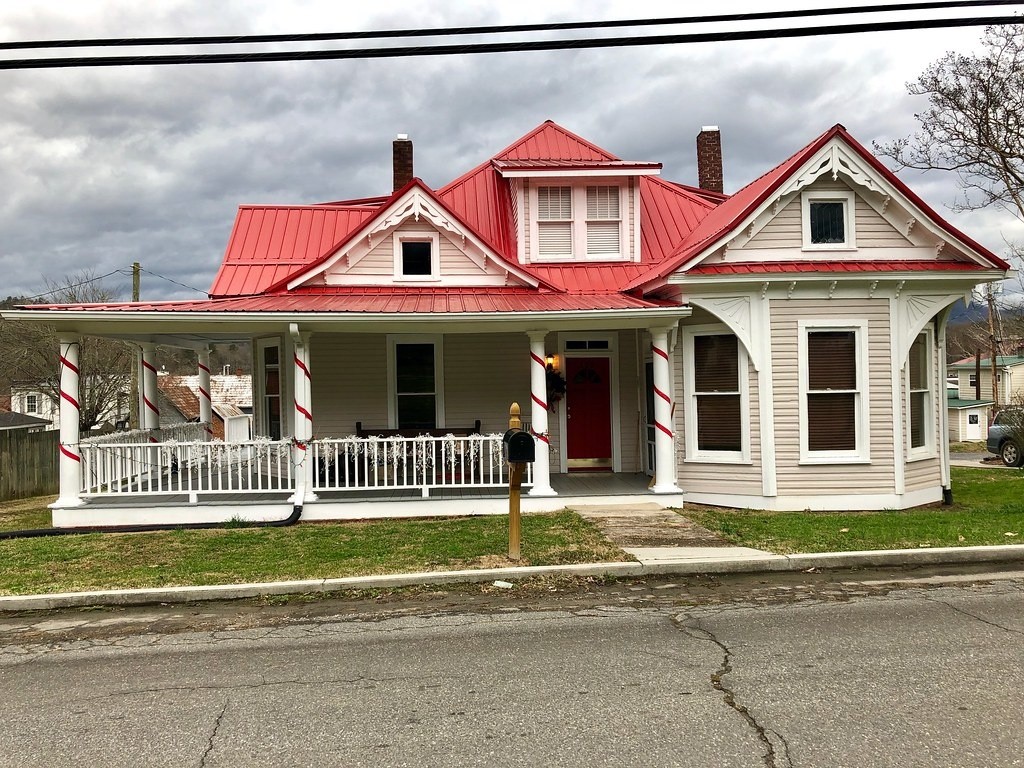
top-left (546, 353), bottom-right (554, 369)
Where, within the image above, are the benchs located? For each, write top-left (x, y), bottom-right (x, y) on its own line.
top-left (355, 420), bottom-right (482, 481)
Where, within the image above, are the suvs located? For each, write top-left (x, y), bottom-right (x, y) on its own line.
top-left (986, 407), bottom-right (1024, 467)
